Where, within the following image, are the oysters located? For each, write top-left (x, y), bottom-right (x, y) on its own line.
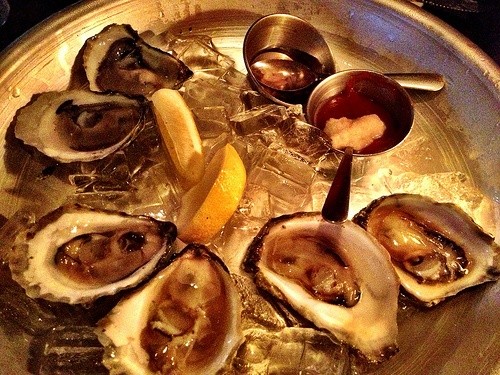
top-left (8, 23), bottom-right (500, 375)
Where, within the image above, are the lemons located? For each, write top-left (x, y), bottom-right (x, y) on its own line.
top-left (151, 87), bottom-right (204, 186)
top-left (176, 142), bottom-right (245, 244)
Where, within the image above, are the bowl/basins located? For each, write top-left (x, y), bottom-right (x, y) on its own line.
top-left (244, 13), bottom-right (336, 106)
top-left (307, 70), bottom-right (414, 156)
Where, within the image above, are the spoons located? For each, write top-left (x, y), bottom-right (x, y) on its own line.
top-left (252, 58), bottom-right (446, 91)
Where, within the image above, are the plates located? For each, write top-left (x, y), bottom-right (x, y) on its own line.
top-left (0, 1), bottom-right (500, 373)
